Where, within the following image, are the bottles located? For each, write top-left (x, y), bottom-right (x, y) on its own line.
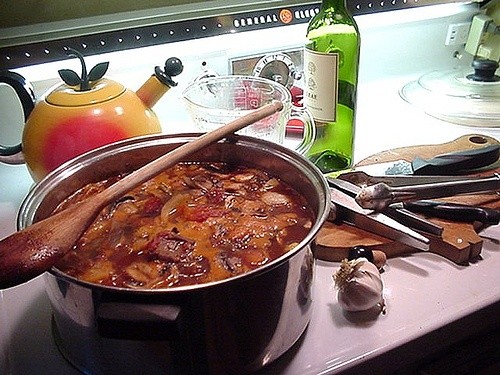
top-left (302, 0), bottom-right (361, 174)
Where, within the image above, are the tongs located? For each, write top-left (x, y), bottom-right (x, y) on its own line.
top-left (336, 171), bottom-right (500, 212)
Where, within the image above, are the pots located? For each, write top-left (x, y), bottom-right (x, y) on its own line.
top-left (15, 131), bottom-right (331, 374)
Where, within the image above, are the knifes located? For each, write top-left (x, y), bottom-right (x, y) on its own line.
top-left (321, 142), bottom-right (500, 180)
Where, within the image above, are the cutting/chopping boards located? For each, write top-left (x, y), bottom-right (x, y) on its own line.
top-left (312, 133), bottom-right (500, 264)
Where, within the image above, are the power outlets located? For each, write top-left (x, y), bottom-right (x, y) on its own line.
top-left (445, 23), bottom-right (470, 45)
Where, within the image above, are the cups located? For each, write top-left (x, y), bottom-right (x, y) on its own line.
top-left (183, 75), bottom-right (316, 156)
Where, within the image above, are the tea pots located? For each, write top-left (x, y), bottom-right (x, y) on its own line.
top-left (0, 48), bottom-right (185, 184)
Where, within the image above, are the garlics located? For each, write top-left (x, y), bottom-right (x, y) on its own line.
top-left (333, 257), bottom-right (389, 316)
